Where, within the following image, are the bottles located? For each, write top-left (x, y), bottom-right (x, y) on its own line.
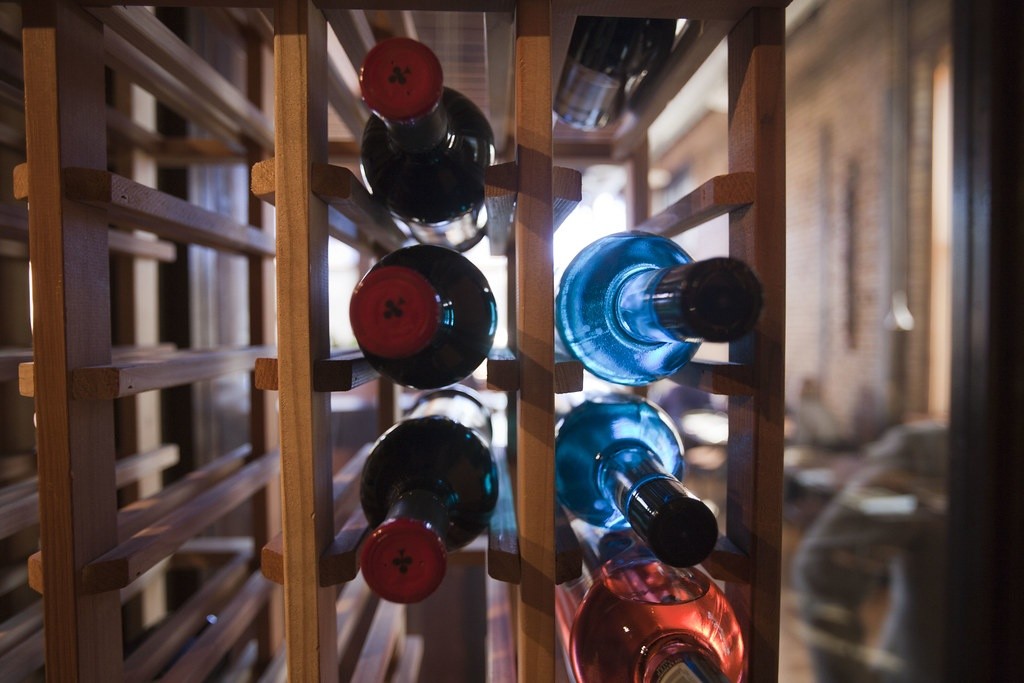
top-left (552, 508), bottom-right (748, 683)
top-left (554, 230), bottom-right (764, 387)
top-left (554, 17), bottom-right (678, 133)
top-left (555, 392), bottom-right (719, 568)
top-left (361, 35), bottom-right (498, 253)
top-left (359, 386), bottom-right (498, 604)
top-left (349, 245), bottom-right (498, 392)
